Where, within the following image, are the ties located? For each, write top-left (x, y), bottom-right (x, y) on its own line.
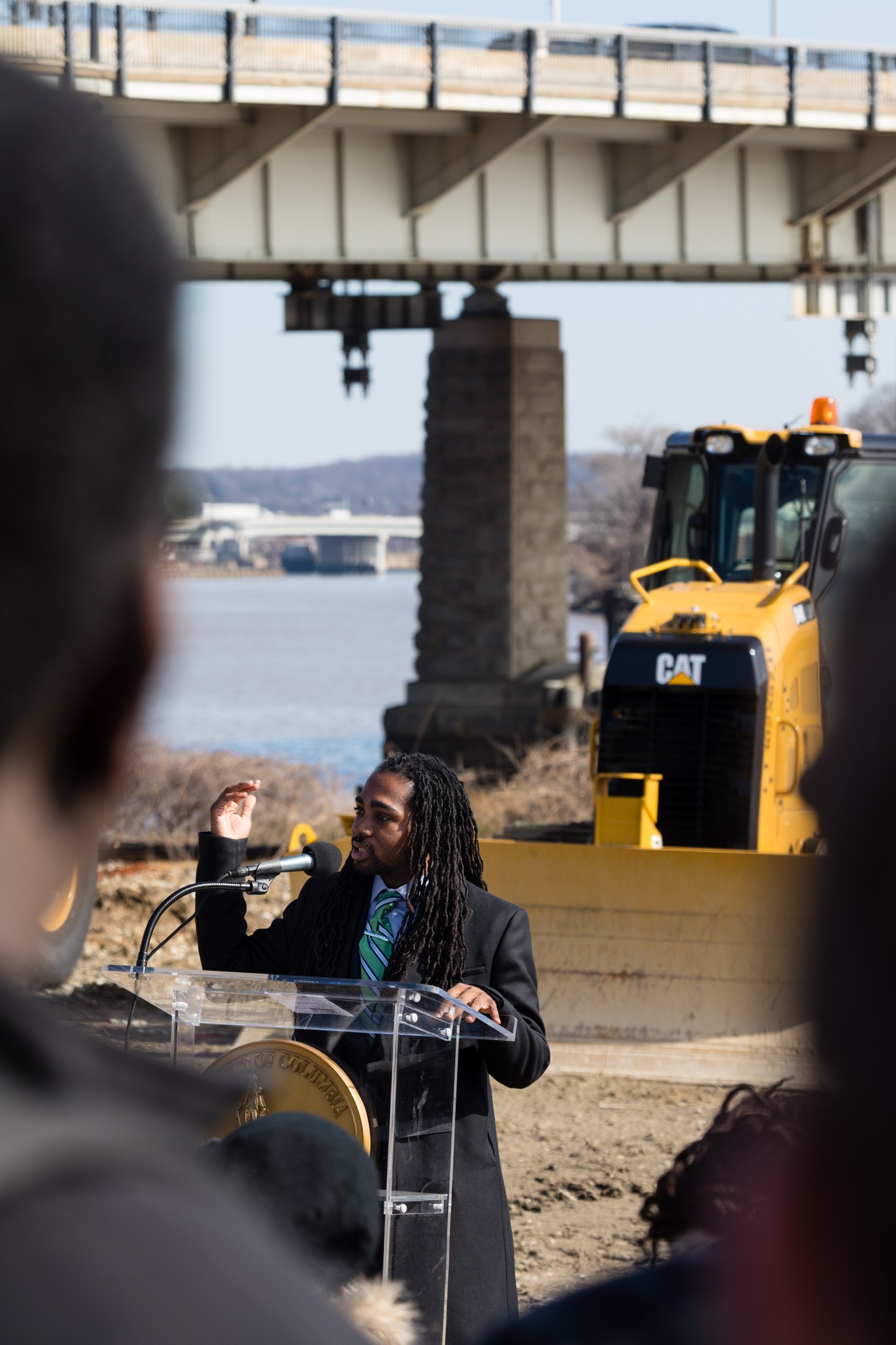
top-left (358, 891), bottom-right (404, 1047)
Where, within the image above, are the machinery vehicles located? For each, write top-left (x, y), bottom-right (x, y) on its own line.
top-left (587, 393), bottom-right (896, 852)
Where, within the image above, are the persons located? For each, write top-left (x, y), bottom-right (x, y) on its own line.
top-left (0, 56), bottom-right (896, 1345)
top-left (191, 752), bottom-right (553, 1345)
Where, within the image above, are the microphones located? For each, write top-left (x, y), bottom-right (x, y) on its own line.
top-left (229, 842), bottom-right (342, 879)
